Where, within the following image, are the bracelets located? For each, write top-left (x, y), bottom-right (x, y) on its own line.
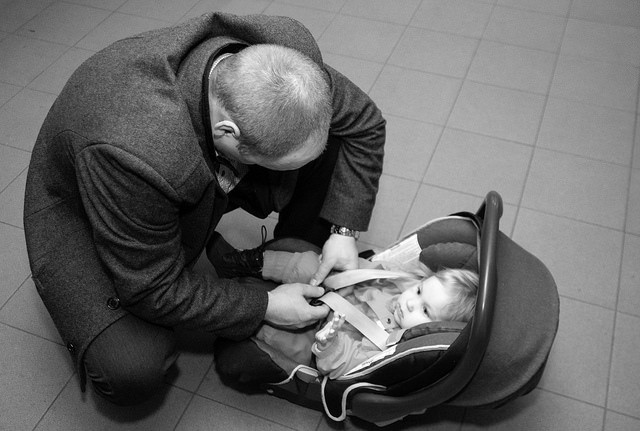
top-left (330, 225), bottom-right (361, 240)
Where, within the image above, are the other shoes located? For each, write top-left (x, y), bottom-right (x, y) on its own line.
top-left (206, 225), bottom-right (261, 279)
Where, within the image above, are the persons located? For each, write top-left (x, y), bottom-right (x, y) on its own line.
top-left (23, 12), bottom-right (387, 405)
top-left (204, 225), bottom-right (481, 376)
